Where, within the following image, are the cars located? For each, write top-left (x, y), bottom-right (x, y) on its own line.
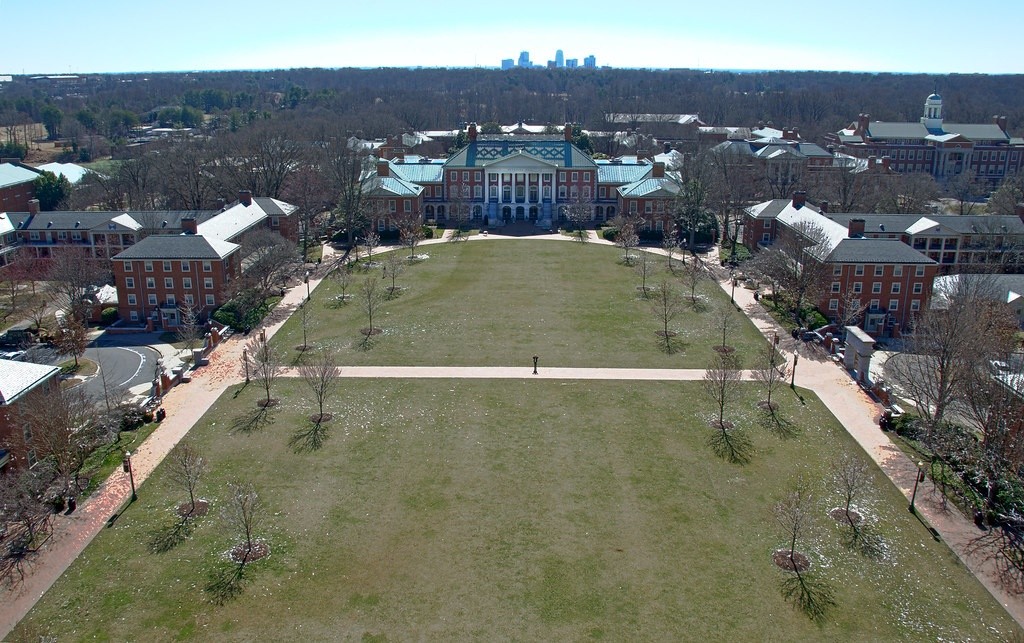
top-left (4, 351), bottom-right (28, 362)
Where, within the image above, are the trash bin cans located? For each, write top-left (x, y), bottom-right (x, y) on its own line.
top-left (721, 259), bottom-right (724, 266)
top-left (244, 325), bottom-right (251, 335)
top-left (753, 292), bottom-right (758, 301)
top-left (879, 417), bottom-right (888, 431)
top-left (68, 497), bottom-right (76, 510)
top-left (318, 256), bottom-right (321, 264)
top-left (156, 408), bottom-right (165, 422)
top-left (974, 511), bottom-right (983, 526)
top-left (792, 329), bottom-right (798, 338)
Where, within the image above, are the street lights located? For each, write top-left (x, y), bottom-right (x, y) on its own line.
top-left (772, 329), bottom-right (778, 353)
top-left (243, 346), bottom-right (250, 383)
top-left (908, 461), bottom-right (924, 514)
top-left (306, 271), bottom-right (311, 301)
top-left (262, 326), bottom-right (267, 348)
top-left (682, 238), bottom-right (686, 264)
top-left (355, 236), bottom-right (359, 262)
top-left (731, 274), bottom-right (736, 304)
top-left (126, 450), bottom-right (138, 503)
top-left (790, 350), bottom-right (799, 389)
